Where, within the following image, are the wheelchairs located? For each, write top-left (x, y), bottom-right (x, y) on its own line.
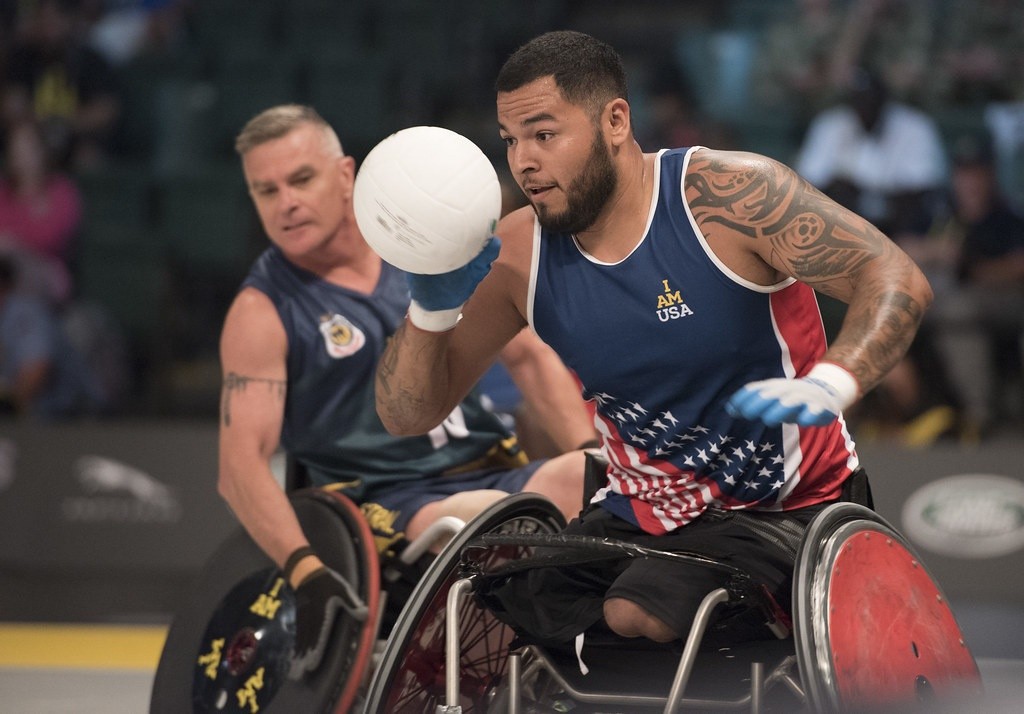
top-left (148, 483), bottom-right (474, 714)
top-left (362, 446), bottom-right (992, 714)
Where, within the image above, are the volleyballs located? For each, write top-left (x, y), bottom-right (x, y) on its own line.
top-left (350, 125), bottom-right (506, 276)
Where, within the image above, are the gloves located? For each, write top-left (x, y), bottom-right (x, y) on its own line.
top-left (283, 568), bottom-right (371, 683)
top-left (725, 358), bottom-right (860, 427)
top-left (406, 238), bottom-right (502, 333)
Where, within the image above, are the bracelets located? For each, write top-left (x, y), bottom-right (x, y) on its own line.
top-left (282, 547), bottom-right (321, 588)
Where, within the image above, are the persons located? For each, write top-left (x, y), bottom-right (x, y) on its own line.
top-left (776, 30), bottom-right (1024, 449)
top-left (218, 103), bottom-right (549, 673)
top-left (374, 31), bottom-right (933, 643)
